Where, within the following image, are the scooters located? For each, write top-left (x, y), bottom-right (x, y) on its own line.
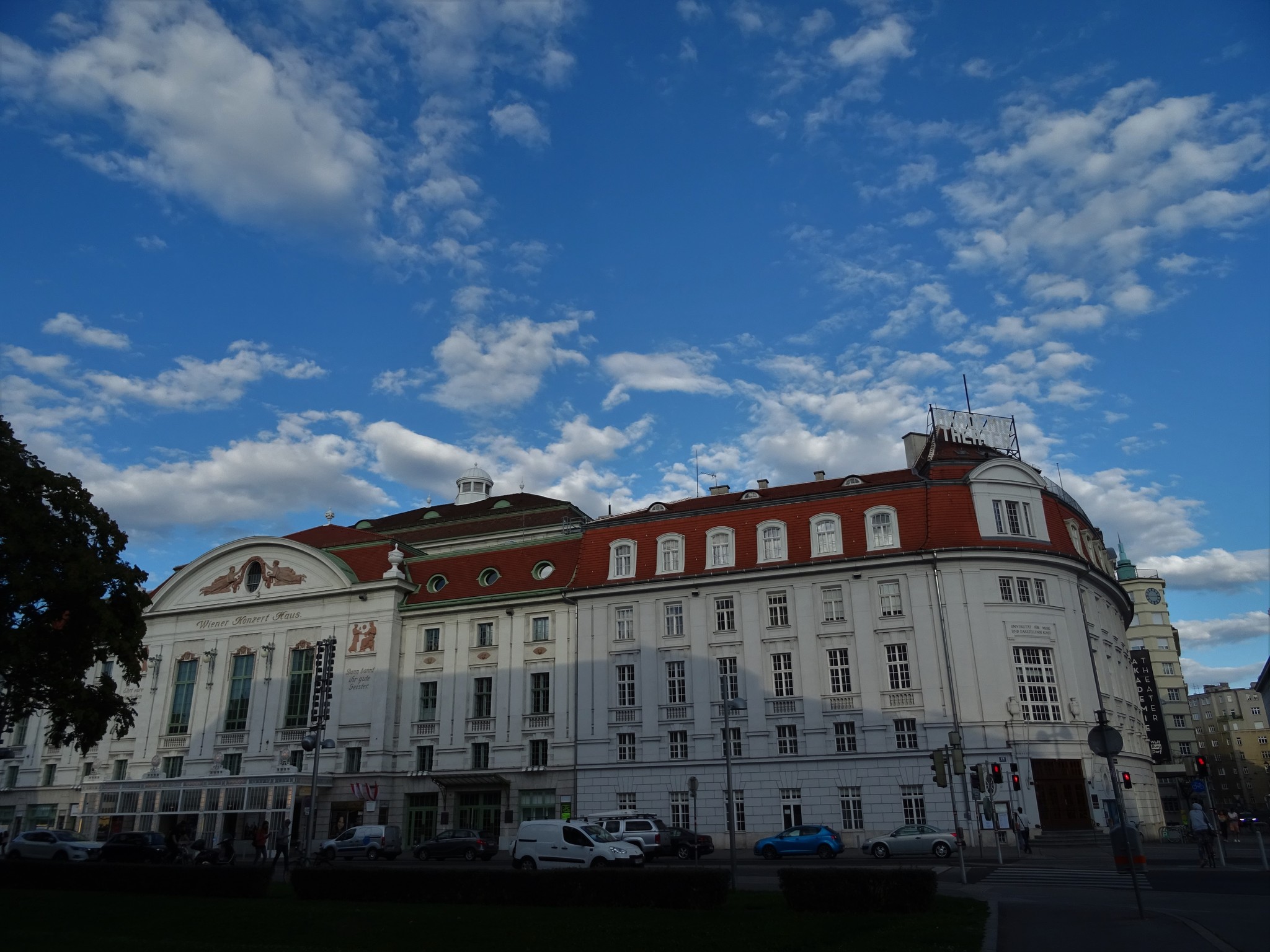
top-left (191, 837), bottom-right (239, 866)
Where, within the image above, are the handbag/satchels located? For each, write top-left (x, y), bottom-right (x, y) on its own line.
top-left (1208, 826), bottom-right (1218, 837)
top-left (1024, 827), bottom-right (1029, 831)
top-left (252, 840), bottom-right (259, 847)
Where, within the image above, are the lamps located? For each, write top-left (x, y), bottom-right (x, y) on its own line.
top-left (147, 653), bottom-right (162, 694)
top-left (527, 766), bottom-right (533, 771)
top-left (852, 571), bottom-right (862, 578)
top-left (1264, 761), bottom-right (1269, 764)
top-left (359, 593), bottom-right (368, 602)
top-left (412, 771), bottom-right (418, 776)
top-left (506, 608), bottom-right (514, 616)
top-left (202, 647), bottom-right (218, 690)
top-left (417, 771), bottom-right (424, 776)
top-left (539, 766), bottom-right (546, 771)
top-left (260, 641), bottom-right (276, 684)
top-left (691, 590), bottom-right (700, 596)
top-left (422, 771), bottom-right (431, 776)
top-left (407, 771), bottom-right (413, 776)
top-left (533, 766), bottom-right (540, 771)
top-left (522, 766), bottom-right (527, 771)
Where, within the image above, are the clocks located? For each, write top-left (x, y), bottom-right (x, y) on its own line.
top-left (1145, 587), bottom-right (1161, 605)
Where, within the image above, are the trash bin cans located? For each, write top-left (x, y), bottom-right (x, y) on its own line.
top-left (1109, 823), bottom-right (1147, 874)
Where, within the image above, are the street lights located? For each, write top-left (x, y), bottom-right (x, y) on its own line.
top-left (301, 638), bottom-right (337, 868)
top-left (722, 674), bottom-right (749, 890)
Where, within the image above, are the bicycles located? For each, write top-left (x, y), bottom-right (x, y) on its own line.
top-left (1166, 820), bottom-right (1193, 843)
top-left (1130, 821), bottom-right (1144, 843)
top-left (285, 847), bottom-right (334, 871)
top-left (1196, 829), bottom-right (1216, 868)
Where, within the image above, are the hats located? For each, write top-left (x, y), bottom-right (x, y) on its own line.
top-left (1230, 807), bottom-right (1234, 811)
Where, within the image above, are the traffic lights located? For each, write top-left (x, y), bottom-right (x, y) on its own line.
top-left (1012, 775), bottom-right (1021, 791)
top-left (928, 750), bottom-right (949, 790)
top-left (991, 763), bottom-right (1004, 784)
top-left (1122, 772), bottom-right (1132, 789)
top-left (1194, 755), bottom-right (1209, 777)
top-left (969, 762), bottom-right (988, 794)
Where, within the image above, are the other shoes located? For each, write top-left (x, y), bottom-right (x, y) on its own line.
top-left (1200, 860), bottom-right (1206, 868)
top-left (1028, 852), bottom-right (1032, 854)
top-left (1238, 840), bottom-right (1240, 842)
top-left (1234, 839), bottom-right (1237, 842)
top-left (1025, 850), bottom-right (1028, 853)
top-left (1223, 839), bottom-right (1228, 842)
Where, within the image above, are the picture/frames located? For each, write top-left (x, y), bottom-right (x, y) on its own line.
top-left (243, 812), bottom-right (261, 840)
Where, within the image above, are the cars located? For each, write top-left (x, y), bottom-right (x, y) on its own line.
top-left (413, 829), bottom-right (499, 861)
top-left (99, 831), bottom-right (167, 865)
top-left (1238, 812), bottom-right (1259, 825)
top-left (6, 829), bottom-right (105, 865)
top-left (659, 826), bottom-right (714, 860)
top-left (862, 823), bottom-right (966, 859)
top-left (753, 825), bottom-right (845, 860)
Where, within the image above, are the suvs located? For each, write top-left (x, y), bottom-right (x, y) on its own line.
top-left (587, 808), bottom-right (672, 858)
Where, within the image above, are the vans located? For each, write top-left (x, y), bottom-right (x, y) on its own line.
top-left (322, 824), bottom-right (402, 861)
top-left (511, 816), bottom-right (645, 870)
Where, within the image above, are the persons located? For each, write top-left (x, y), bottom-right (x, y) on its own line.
top-left (165, 820), bottom-right (192, 858)
top-left (331, 815), bottom-right (349, 837)
top-left (0, 831), bottom-right (9, 855)
top-left (1011, 807), bottom-right (1032, 855)
top-left (269, 819), bottom-right (292, 875)
top-left (1187, 801), bottom-right (1241, 868)
top-left (97, 823), bottom-right (110, 833)
top-left (254, 821), bottom-right (272, 865)
top-left (245, 823), bottom-right (256, 840)
